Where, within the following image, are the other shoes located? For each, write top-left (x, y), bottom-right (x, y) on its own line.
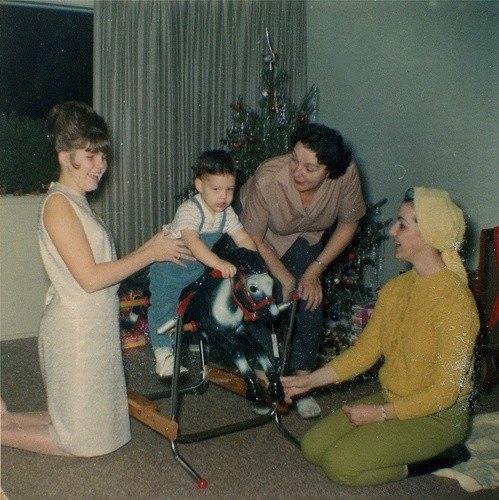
top-left (251, 401), bottom-right (270, 415)
top-left (443, 442), bottom-right (470, 464)
top-left (297, 397), bottom-right (320, 418)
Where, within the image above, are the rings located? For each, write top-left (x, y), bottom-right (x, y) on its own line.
top-left (177, 253), bottom-right (179, 259)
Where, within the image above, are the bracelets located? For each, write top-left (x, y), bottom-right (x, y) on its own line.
top-left (315, 259), bottom-right (326, 269)
top-left (380, 405), bottom-right (388, 421)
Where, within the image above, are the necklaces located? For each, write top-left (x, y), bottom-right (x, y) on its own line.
top-left (440, 266), bottom-right (446, 273)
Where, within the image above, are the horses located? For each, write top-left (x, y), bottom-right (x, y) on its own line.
top-left (128, 249), bottom-right (286, 415)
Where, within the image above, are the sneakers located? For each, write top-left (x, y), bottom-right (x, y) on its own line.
top-left (155, 346), bottom-right (188, 379)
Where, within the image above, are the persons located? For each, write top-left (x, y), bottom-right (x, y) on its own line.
top-left (279, 186), bottom-right (480, 485)
top-left (0, 100), bottom-right (195, 458)
top-left (236, 123), bottom-right (366, 418)
top-left (147, 150), bottom-right (258, 378)
